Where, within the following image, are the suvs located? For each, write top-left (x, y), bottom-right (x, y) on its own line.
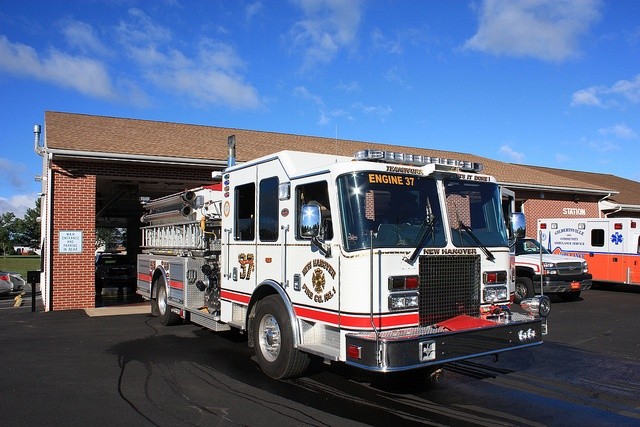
top-left (95, 250), bottom-right (137, 293)
top-left (514, 236), bottom-right (592, 304)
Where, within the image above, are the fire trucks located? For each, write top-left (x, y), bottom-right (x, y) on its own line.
top-left (135, 135), bottom-right (551, 379)
top-left (537, 217), bottom-right (640, 285)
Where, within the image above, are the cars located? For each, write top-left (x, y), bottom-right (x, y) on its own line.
top-left (0, 271), bottom-right (13, 294)
